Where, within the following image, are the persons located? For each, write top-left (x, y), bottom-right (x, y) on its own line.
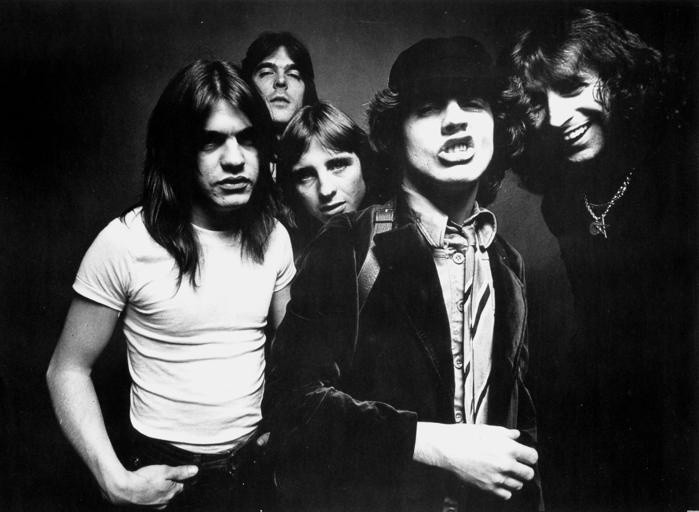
top-left (243, 31), bottom-right (320, 181)
top-left (46, 55), bottom-right (301, 509)
top-left (496, 5), bottom-right (699, 508)
top-left (274, 102), bottom-right (392, 267)
top-left (258, 37), bottom-right (550, 511)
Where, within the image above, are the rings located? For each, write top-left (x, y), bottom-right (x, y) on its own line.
top-left (498, 473), bottom-right (509, 485)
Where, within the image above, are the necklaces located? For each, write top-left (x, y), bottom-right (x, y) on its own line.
top-left (581, 160), bottom-right (637, 241)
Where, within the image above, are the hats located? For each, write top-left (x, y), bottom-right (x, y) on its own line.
top-left (388, 36), bottom-right (497, 93)
